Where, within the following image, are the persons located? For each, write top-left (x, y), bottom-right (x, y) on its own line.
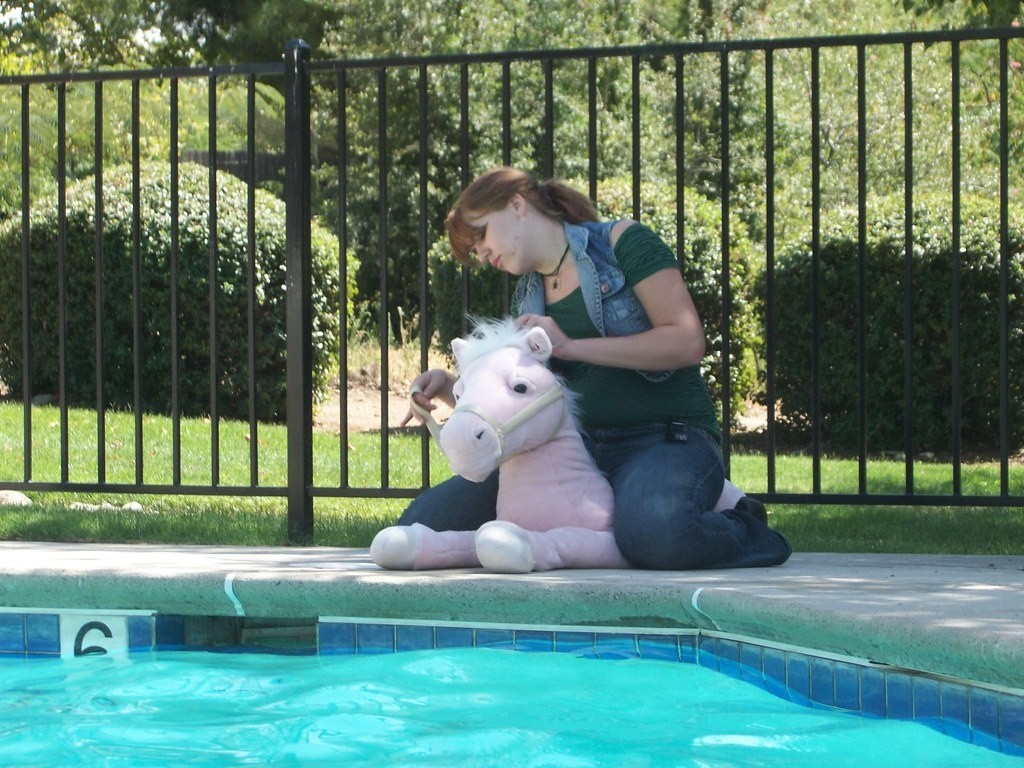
top-left (396, 169), bottom-right (792, 571)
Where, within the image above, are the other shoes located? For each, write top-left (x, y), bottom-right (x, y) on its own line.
top-left (733, 496), bottom-right (768, 527)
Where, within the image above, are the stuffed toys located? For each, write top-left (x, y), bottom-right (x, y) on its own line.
top-left (370, 315), bottom-right (746, 574)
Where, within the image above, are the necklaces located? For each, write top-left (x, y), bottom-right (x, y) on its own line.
top-left (541, 243), bottom-right (571, 291)
